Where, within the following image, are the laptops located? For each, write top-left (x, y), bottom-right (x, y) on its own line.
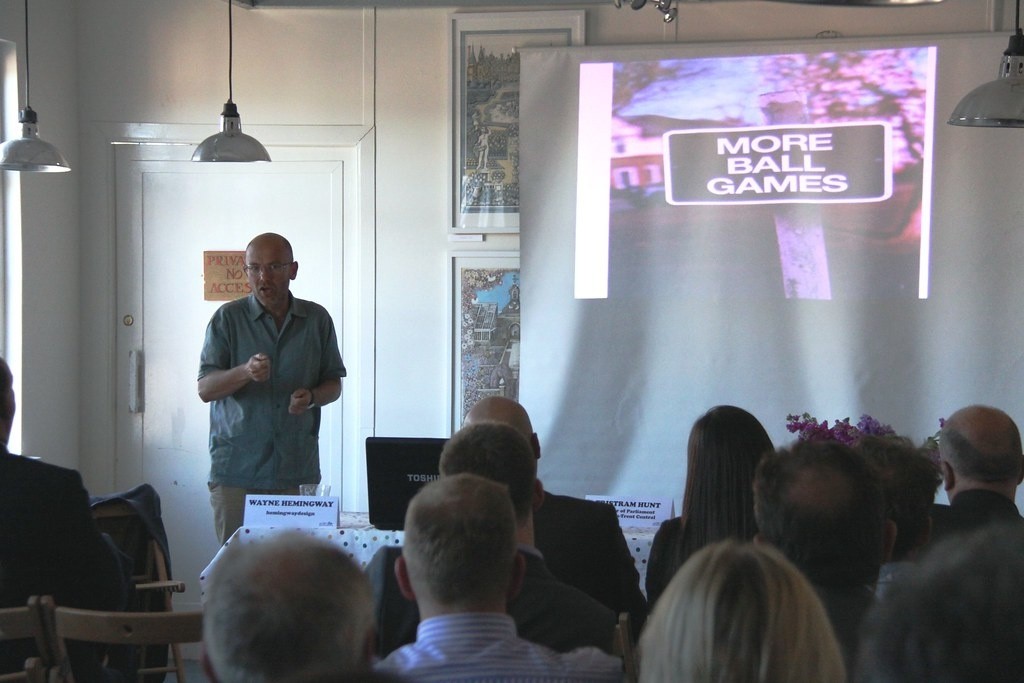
top-left (364, 437), bottom-right (451, 529)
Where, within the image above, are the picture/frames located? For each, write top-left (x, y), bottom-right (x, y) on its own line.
top-left (449, 10), bottom-right (585, 235)
top-left (448, 251), bottom-right (524, 432)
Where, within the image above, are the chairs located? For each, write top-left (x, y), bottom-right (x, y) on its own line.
top-left (1, 483), bottom-right (215, 683)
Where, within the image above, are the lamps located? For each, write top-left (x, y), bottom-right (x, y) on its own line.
top-left (948, 0), bottom-right (1024, 129)
top-left (615, 0), bottom-right (677, 23)
top-left (190, 1), bottom-right (271, 164)
top-left (0, 0), bottom-right (71, 173)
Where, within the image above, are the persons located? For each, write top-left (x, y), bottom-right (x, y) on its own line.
top-left (639, 539), bottom-right (844, 683)
top-left (368, 422), bottom-right (617, 656)
top-left (198, 233), bottom-right (347, 547)
top-left (753, 406), bottom-right (1024, 683)
top-left (374, 473), bottom-right (622, 683)
top-left (198, 531), bottom-right (374, 683)
top-left (0, 358), bottom-right (135, 675)
top-left (644, 404), bottom-right (775, 599)
top-left (465, 396), bottom-right (648, 640)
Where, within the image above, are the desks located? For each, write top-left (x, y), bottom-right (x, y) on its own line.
top-left (200, 513), bottom-right (655, 620)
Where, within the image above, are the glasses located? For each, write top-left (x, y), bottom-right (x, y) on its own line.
top-left (248, 263), bottom-right (292, 274)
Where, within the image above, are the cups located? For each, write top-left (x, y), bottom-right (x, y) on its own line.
top-left (315, 484), bottom-right (331, 496)
top-left (299, 484), bottom-right (317, 496)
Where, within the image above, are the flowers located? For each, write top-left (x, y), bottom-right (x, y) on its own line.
top-left (786, 412), bottom-right (948, 498)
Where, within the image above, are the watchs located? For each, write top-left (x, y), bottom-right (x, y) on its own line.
top-left (308, 388), bottom-right (314, 409)
